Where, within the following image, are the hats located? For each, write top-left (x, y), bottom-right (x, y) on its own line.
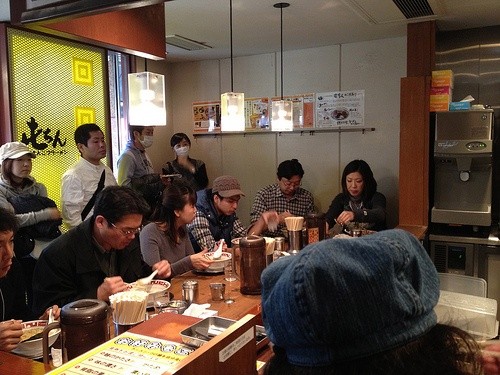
top-left (212, 176), bottom-right (246, 198)
top-left (0, 142), bottom-right (37, 162)
top-left (260, 231), bottom-right (440, 367)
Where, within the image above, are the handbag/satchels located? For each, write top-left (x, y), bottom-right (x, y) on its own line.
top-left (7, 195), bottom-right (62, 238)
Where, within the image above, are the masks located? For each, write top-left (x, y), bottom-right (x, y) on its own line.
top-left (175, 146), bottom-right (189, 156)
top-left (137, 135), bottom-right (153, 149)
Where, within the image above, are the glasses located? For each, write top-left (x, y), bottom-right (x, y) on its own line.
top-left (104, 217), bottom-right (144, 235)
top-left (279, 177), bottom-right (302, 188)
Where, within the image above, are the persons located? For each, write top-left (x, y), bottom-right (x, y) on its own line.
top-left (261, 229), bottom-right (481, 375)
top-left (161, 133), bottom-right (209, 191)
top-left (140, 183), bottom-right (228, 279)
top-left (187, 176), bottom-right (280, 251)
top-left (61, 124), bottom-right (118, 230)
top-left (326, 160), bottom-right (388, 237)
top-left (0, 141), bottom-right (61, 312)
top-left (0, 208), bottom-right (61, 351)
top-left (29, 186), bottom-right (171, 308)
top-left (117, 126), bottom-right (167, 189)
top-left (250, 158), bottom-right (321, 237)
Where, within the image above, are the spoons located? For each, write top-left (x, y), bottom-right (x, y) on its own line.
top-left (214, 238), bottom-right (225, 259)
top-left (48, 308), bottom-right (57, 336)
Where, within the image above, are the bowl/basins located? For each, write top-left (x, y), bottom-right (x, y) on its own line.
top-left (123, 279), bottom-right (171, 304)
top-left (11, 319), bottom-right (62, 356)
top-left (202, 252), bottom-right (232, 272)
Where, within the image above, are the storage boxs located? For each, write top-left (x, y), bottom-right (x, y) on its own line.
top-left (431, 70), bottom-right (455, 81)
top-left (429, 95), bottom-right (452, 104)
top-left (435, 286), bottom-right (499, 340)
top-left (431, 78), bottom-right (454, 89)
top-left (430, 87), bottom-right (453, 95)
top-left (429, 104), bottom-right (449, 112)
top-left (450, 102), bottom-right (471, 110)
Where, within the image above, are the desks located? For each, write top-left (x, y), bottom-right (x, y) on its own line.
top-left (0, 261), bottom-right (275, 375)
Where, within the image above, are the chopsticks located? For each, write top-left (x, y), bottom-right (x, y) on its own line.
top-left (284, 216), bottom-right (304, 231)
top-left (109, 290), bottom-right (148, 324)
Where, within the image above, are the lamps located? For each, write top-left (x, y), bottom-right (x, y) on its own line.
top-left (270, 1), bottom-right (294, 133)
top-left (128, 53), bottom-right (169, 128)
top-left (218, 0), bottom-right (247, 134)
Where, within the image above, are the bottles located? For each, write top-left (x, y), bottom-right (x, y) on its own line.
top-left (238, 235), bottom-right (266, 295)
top-left (182, 281), bottom-right (199, 305)
top-left (305, 213), bottom-right (325, 245)
top-left (275, 237), bottom-right (285, 251)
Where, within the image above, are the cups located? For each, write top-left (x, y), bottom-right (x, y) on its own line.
top-left (210, 284), bottom-right (226, 300)
top-left (112, 319), bottom-right (144, 337)
top-left (41, 299), bottom-right (110, 372)
top-left (224, 260), bottom-right (237, 281)
top-left (287, 230), bottom-right (303, 255)
top-left (266, 253), bottom-right (273, 266)
